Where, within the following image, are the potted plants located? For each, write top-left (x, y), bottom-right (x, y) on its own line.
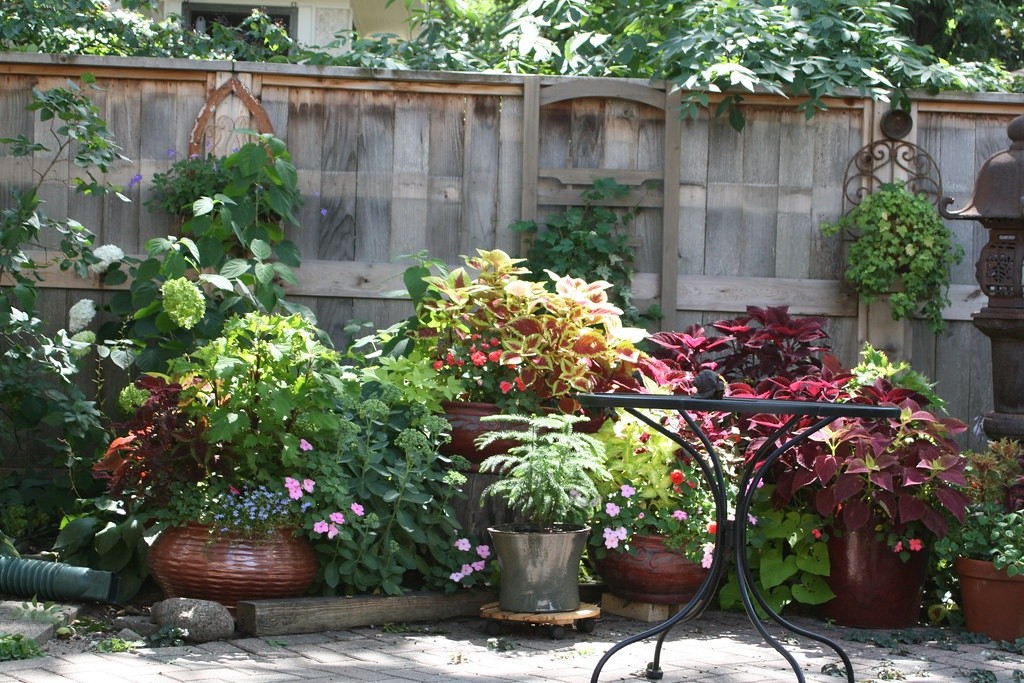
top-left (474, 413), bottom-right (610, 612)
top-left (957, 510), bottom-right (1024, 643)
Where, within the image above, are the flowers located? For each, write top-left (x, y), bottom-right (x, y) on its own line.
top-left (604, 433), bottom-right (718, 570)
top-left (429, 537), bottom-right (495, 592)
top-left (813, 527), bottom-right (922, 553)
top-left (215, 438), bottom-right (364, 540)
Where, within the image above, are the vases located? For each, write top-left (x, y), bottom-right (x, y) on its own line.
top-left (147, 522), bottom-right (320, 623)
top-left (586, 526), bottom-right (711, 602)
top-left (809, 514), bottom-right (929, 629)
